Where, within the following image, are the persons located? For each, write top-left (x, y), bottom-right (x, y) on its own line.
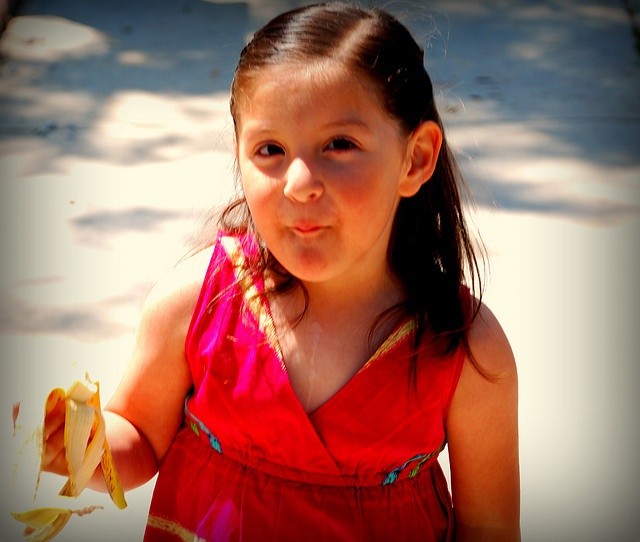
top-left (12, 1), bottom-right (521, 542)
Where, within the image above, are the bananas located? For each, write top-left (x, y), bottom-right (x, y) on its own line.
top-left (11, 356), bottom-right (127, 541)
